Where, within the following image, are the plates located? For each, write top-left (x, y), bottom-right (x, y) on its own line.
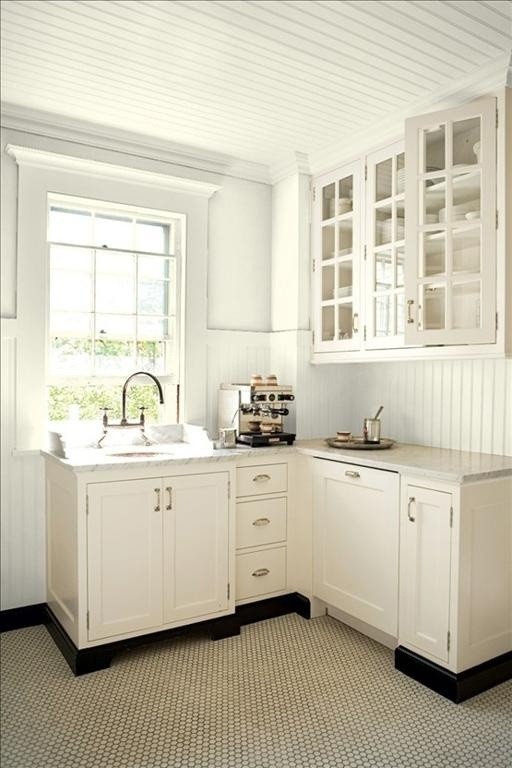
top-left (325, 437), bottom-right (394, 450)
top-left (396, 165), bottom-right (443, 194)
top-left (380, 204), bottom-right (480, 246)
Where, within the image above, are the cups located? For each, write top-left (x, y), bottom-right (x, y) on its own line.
top-left (335, 431), bottom-right (351, 442)
top-left (364, 418), bottom-right (380, 443)
top-left (217, 428), bottom-right (236, 449)
top-left (251, 374), bottom-right (277, 385)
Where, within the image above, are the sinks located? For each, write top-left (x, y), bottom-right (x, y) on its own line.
top-left (104, 451), bottom-right (169, 456)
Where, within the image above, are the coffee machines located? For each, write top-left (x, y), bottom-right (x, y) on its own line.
top-left (218, 381), bottom-right (298, 445)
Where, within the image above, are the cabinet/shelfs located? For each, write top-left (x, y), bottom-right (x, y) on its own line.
top-left (400, 83), bottom-right (512, 364)
top-left (235, 450), bottom-right (299, 611)
top-left (308, 129), bottom-right (429, 365)
top-left (37, 446), bottom-right (242, 678)
top-left (297, 451), bottom-right (401, 651)
top-left (398, 468), bottom-right (511, 679)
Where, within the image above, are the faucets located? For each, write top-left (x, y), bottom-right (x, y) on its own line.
top-left (122, 372), bottom-right (163, 420)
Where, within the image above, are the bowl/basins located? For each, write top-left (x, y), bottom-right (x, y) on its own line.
top-left (459, 248), bottom-right (479, 268)
top-left (334, 286), bottom-right (352, 296)
top-left (329, 197), bottom-right (352, 218)
